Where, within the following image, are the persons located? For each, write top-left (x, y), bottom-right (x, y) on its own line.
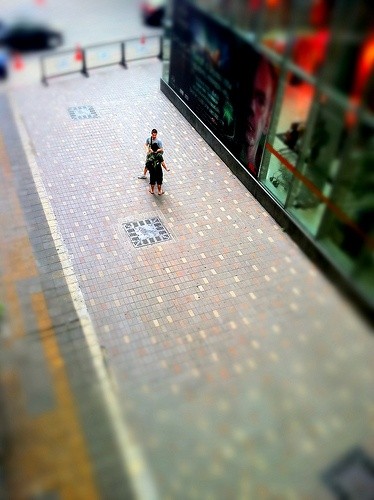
top-left (239, 61), bottom-right (278, 171)
top-left (137, 128), bottom-right (164, 179)
top-left (147, 143), bottom-right (170, 194)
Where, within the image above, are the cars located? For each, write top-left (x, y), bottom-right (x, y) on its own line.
top-left (1, 25), bottom-right (62, 54)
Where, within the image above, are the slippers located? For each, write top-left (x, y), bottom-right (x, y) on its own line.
top-left (149, 189), bottom-right (154, 194)
top-left (159, 190), bottom-right (165, 195)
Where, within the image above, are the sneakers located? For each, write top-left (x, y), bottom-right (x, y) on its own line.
top-left (138, 175), bottom-right (146, 179)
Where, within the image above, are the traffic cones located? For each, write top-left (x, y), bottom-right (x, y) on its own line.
top-left (14, 52), bottom-right (22, 69)
top-left (75, 42), bottom-right (83, 61)
